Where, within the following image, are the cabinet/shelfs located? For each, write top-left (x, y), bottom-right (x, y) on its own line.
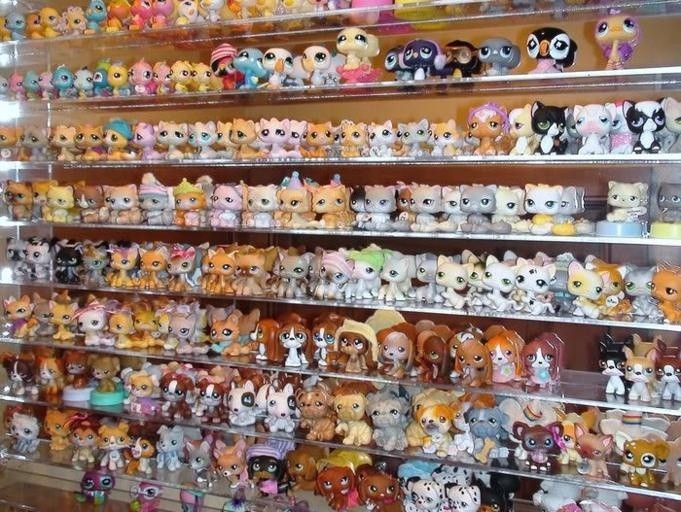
top-left (0, 4), bottom-right (681, 512)
